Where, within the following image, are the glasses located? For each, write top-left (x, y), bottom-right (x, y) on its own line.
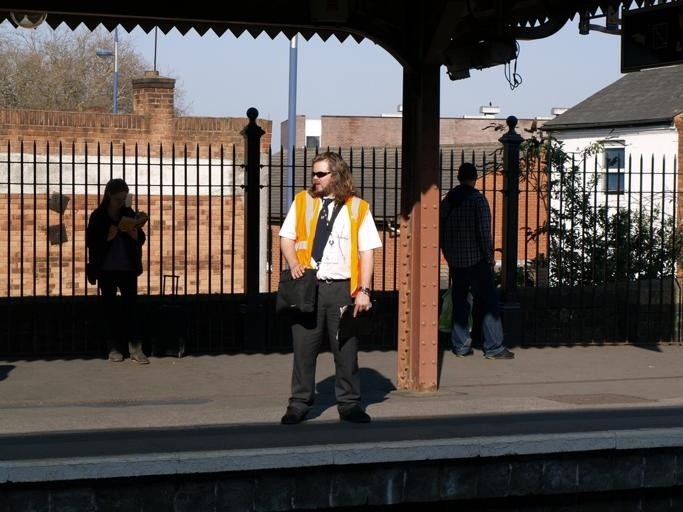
top-left (311, 170), bottom-right (331, 178)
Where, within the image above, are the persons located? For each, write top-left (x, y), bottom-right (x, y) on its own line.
top-left (278, 153), bottom-right (383, 425)
top-left (440, 162), bottom-right (514, 359)
top-left (86, 179), bottom-right (151, 365)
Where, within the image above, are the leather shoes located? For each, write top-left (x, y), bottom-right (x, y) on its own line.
top-left (339, 407), bottom-right (373, 423)
top-left (130, 350), bottom-right (150, 365)
top-left (280, 404), bottom-right (310, 424)
top-left (107, 349), bottom-right (124, 362)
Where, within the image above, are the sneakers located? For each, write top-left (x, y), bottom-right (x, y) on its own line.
top-left (485, 348), bottom-right (516, 360)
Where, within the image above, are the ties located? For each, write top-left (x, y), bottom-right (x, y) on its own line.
top-left (311, 199), bottom-right (333, 262)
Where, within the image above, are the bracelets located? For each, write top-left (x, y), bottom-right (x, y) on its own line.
top-left (361, 287), bottom-right (370, 292)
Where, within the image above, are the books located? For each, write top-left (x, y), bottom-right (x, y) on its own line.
top-left (118, 216), bottom-right (149, 232)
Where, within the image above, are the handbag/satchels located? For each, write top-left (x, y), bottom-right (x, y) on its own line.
top-left (82, 259), bottom-right (100, 286)
top-left (274, 266), bottom-right (317, 315)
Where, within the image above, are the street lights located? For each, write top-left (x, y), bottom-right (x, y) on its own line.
top-left (94, 27), bottom-right (118, 114)
